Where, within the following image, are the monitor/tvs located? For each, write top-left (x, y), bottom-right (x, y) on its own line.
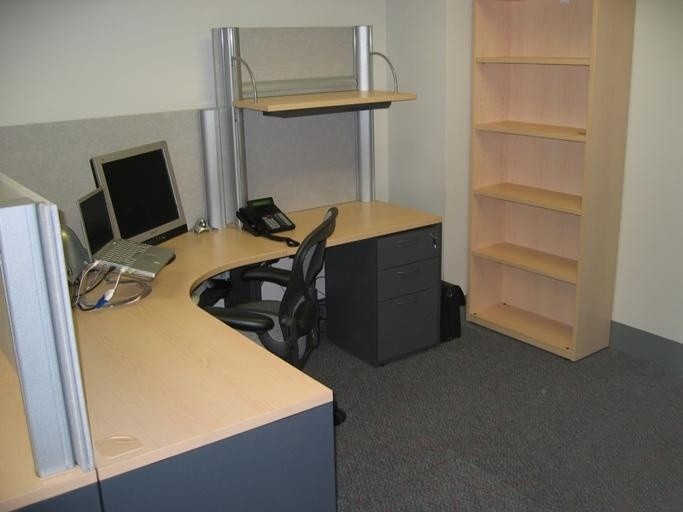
top-left (90, 139), bottom-right (188, 245)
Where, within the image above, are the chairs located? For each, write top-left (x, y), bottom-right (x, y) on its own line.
top-left (197, 207), bottom-right (346, 428)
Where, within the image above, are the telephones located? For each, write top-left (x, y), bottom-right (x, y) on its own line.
top-left (236, 197), bottom-right (295, 236)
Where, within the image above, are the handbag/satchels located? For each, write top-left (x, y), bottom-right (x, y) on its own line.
top-left (440, 277), bottom-right (466, 341)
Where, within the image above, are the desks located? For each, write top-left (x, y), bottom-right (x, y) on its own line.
top-left (1, 200), bottom-right (444, 511)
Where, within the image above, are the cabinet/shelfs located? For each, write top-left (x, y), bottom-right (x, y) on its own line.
top-left (219, 27), bottom-right (418, 230)
top-left (466, 1), bottom-right (636, 363)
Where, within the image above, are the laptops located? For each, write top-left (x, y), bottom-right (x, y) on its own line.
top-left (77, 187), bottom-right (175, 279)
